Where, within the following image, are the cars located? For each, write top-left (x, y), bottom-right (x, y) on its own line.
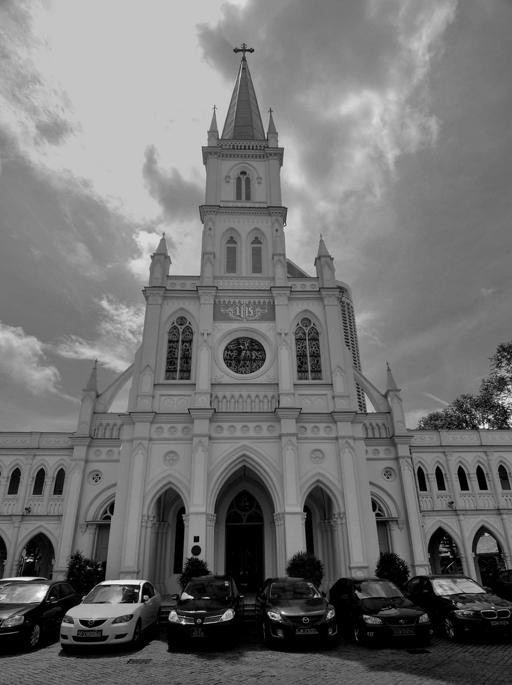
top-left (403, 576), bottom-right (511, 640)
top-left (256, 577), bottom-right (338, 642)
top-left (0, 575), bottom-right (76, 651)
top-left (329, 577), bottom-right (429, 641)
top-left (60, 579), bottom-right (160, 646)
top-left (169, 574), bottom-right (243, 638)
